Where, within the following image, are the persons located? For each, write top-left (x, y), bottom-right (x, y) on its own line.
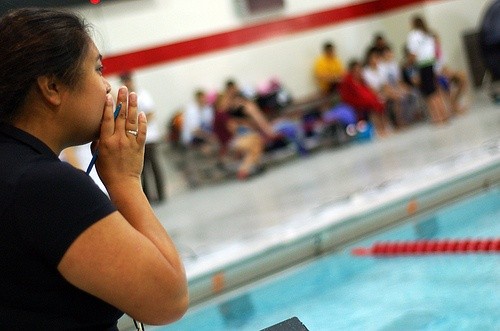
top-left (180, 1), bottom-right (500, 185)
top-left (0, 7), bottom-right (190, 331)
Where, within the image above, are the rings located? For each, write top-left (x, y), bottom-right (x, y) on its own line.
top-left (126, 129), bottom-right (137, 136)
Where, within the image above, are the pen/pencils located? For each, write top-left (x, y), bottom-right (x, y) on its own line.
top-left (83, 101), bottom-right (125, 176)
